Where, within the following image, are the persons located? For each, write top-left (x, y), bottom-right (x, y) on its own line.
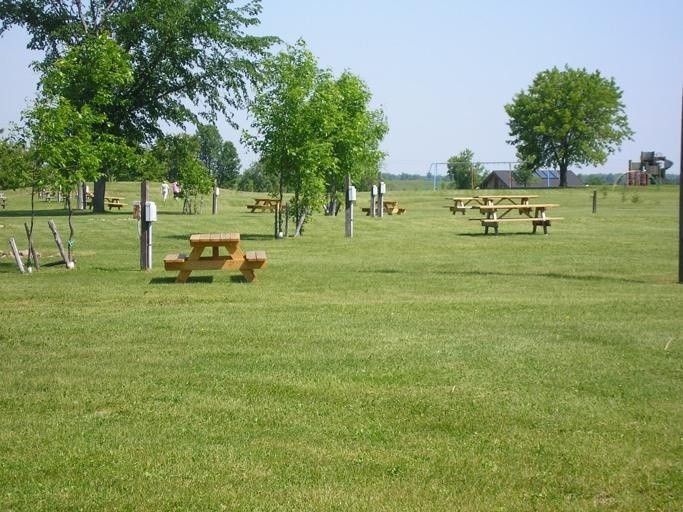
top-left (171, 181), bottom-right (181, 202)
top-left (160, 181), bottom-right (170, 202)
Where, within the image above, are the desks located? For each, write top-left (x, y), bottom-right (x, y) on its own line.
top-left (163, 231), bottom-right (266, 281)
top-left (362, 200), bottom-right (407, 217)
top-left (87, 195), bottom-right (129, 211)
top-left (246, 197), bottom-right (292, 215)
top-left (443, 195), bottom-right (563, 236)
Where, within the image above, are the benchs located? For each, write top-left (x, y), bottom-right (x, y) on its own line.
top-left (480, 217), bottom-right (566, 235)
